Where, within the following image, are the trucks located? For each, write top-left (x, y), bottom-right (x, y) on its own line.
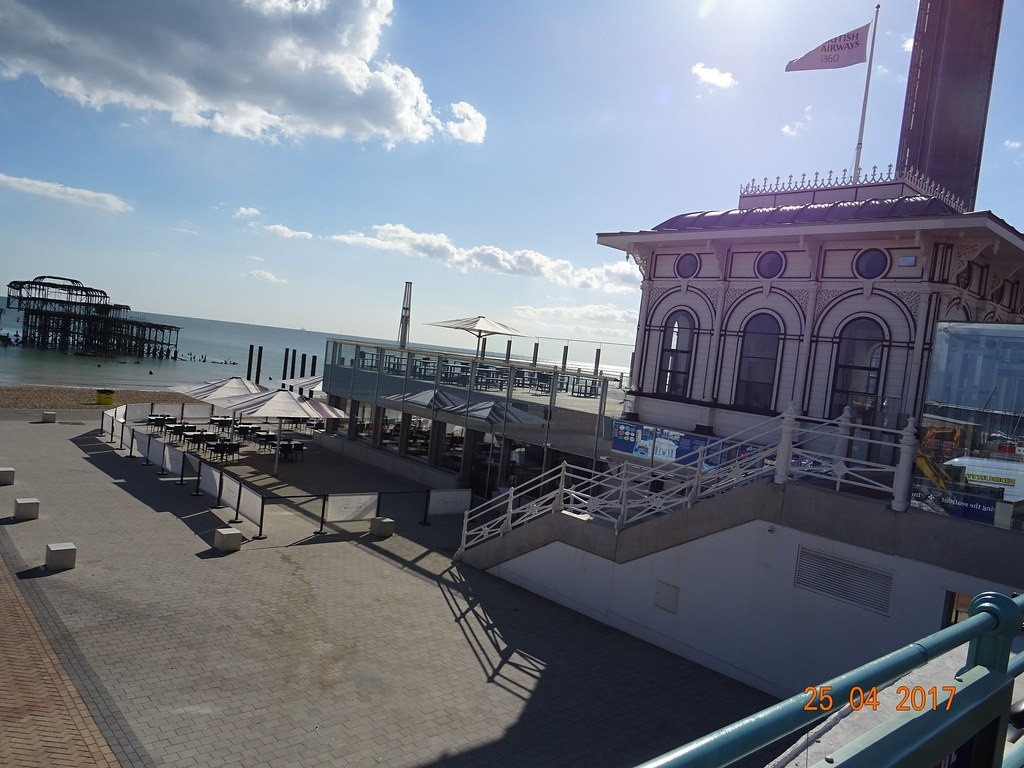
top-left (921, 414), bottom-right (1018, 462)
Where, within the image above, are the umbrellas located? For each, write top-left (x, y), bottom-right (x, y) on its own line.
top-left (166, 374), bottom-right (350, 475)
top-left (421, 316), bottom-right (530, 358)
top-left (379, 388), bottom-right (552, 432)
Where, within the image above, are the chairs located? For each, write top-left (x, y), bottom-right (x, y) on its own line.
top-left (149, 414), bottom-right (305, 463)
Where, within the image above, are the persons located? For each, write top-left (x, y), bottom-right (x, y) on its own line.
top-left (540, 406), bottom-right (550, 429)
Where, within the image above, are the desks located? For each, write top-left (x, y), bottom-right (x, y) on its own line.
top-left (148, 417), bottom-right (175, 432)
top-left (165, 424), bottom-right (194, 441)
top-left (212, 418), bottom-right (239, 430)
top-left (234, 425), bottom-right (259, 433)
top-left (254, 431), bottom-right (276, 449)
top-left (184, 432), bottom-right (215, 449)
top-left (206, 441), bottom-right (239, 462)
top-left (270, 440), bottom-right (301, 455)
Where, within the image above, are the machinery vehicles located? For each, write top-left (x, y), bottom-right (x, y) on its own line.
top-left (914, 421), bottom-right (969, 510)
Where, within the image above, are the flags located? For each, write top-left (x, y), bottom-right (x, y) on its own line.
top-left (785, 22), bottom-right (871, 72)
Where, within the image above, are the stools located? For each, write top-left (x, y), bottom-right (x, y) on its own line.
top-left (361, 352), bottom-right (601, 399)
